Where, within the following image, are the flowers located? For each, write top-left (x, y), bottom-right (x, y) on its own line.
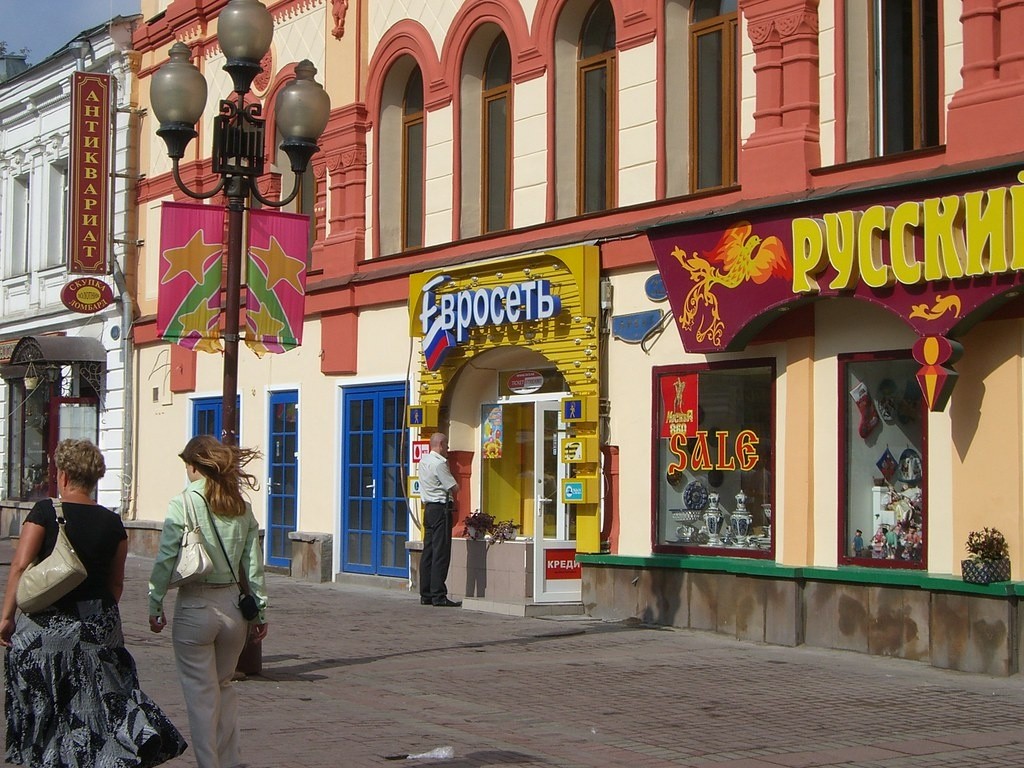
top-left (965, 525), bottom-right (1010, 563)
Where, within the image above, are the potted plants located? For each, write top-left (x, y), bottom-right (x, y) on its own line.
top-left (460, 509), bottom-right (496, 542)
top-left (488, 518), bottom-right (523, 545)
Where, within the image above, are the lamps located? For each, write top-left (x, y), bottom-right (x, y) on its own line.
top-left (45, 364), bottom-right (62, 383)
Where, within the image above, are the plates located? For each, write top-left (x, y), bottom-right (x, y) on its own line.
top-left (684, 481), bottom-right (707, 509)
top-left (667, 469), bottom-right (682, 485)
top-left (698, 405), bottom-right (705, 425)
top-left (708, 468), bottom-right (723, 487)
top-left (708, 427), bottom-right (720, 447)
top-left (688, 438), bottom-right (700, 455)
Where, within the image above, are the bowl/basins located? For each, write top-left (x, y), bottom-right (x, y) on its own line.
top-left (874, 477), bottom-right (884, 486)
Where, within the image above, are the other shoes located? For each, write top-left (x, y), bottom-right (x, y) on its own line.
top-left (421, 596), bottom-right (432, 604)
top-left (431, 598), bottom-right (462, 606)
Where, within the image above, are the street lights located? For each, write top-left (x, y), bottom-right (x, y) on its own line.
top-left (146, 1), bottom-right (332, 678)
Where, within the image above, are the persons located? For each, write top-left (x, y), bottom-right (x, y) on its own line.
top-left (418, 433), bottom-right (460, 606)
top-left (147, 434), bottom-right (268, 768)
top-left (852, 483), bottom-right (922, 561)
top-left (0, 439), bottom-right (189, 768)
top-left (22, 462), bottom-right (43, 498)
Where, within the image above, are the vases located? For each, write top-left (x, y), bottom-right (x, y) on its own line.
top-left (961, 559), bottom-right (1011, 586)
top-left (24, 377), bottom-right (38, 390)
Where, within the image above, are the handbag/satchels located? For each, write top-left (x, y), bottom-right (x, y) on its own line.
top-left (238, 593), bottom-right (259, 620)
top-left (15, 497), bottom-right (87, 614)
top-left (166, 492), bottom-right (214, 589)
